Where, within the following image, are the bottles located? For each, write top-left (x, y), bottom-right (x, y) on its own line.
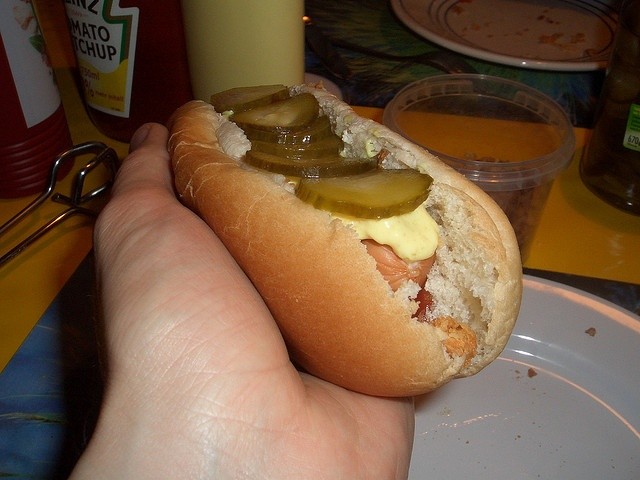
top-left (64, 2), bottom-right (187, 142)
top-left (579, 0), bottom-right (640, 215)
top-left (0, 0), bottom-right (76, 201)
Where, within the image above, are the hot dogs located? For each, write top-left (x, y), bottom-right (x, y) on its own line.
top-left (170, 79), bottom-right (523, 397)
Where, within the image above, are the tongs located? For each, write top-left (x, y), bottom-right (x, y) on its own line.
top-left (0, 141), bottom-right (121, 270)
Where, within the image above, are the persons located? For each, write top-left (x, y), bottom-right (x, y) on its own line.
top-left (68, 122), bottom-right (416, 476)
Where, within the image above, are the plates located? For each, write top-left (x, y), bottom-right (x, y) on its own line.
top-left (407, 274), bottom-right (639, 479)
top-left (393, 2), bottom-right (617, 72)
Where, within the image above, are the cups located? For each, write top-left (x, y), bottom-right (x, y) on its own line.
top-left (384, 73), bottom-right (576, 264)
